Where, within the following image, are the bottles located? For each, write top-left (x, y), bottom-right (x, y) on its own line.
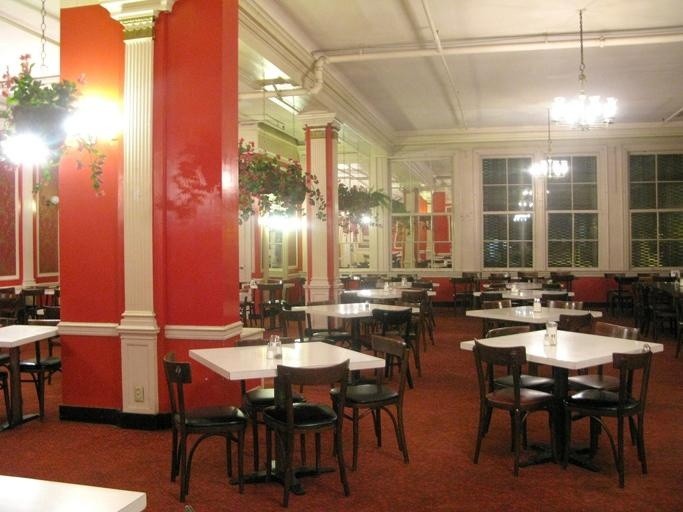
top-left (533, 298), bottom-right (541, 312)
top-left (273, 343), bottom-right (282, 360)
top-left (363, 299), bottom-right (369, 312)
top-left (544, 334), bottom-right (552, 345)
top-left (266, 343), bottom-right (274, 360)
top-left (383, 282), bottom-right (389, 292)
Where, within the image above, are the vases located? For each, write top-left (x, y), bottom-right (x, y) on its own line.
top-left (12, 103), bottom-right (72, 149)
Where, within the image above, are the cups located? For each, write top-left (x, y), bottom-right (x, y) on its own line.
top-left (270, 334), bottom-right (280, 359)
top-left (546, 321), bottom-right (558, 344)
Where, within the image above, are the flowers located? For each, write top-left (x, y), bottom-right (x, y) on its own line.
top-left (239, 137), bottom-right (330, 226)
top-left (1, 53), bottom-right (109, 197)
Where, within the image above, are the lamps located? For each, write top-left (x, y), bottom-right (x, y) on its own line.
top-left (547, 8), bottom-right (619, 129)
top-left (526, 109), bottom-right (569, 179)
top-left (513, 187), bottom-right (551, 223)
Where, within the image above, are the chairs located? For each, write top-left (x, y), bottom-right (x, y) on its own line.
top-left (0, 285), bottom-right (61, 433)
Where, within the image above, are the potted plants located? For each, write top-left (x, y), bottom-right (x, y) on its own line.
top-left (338, 179), bottom-right (392, 234)
